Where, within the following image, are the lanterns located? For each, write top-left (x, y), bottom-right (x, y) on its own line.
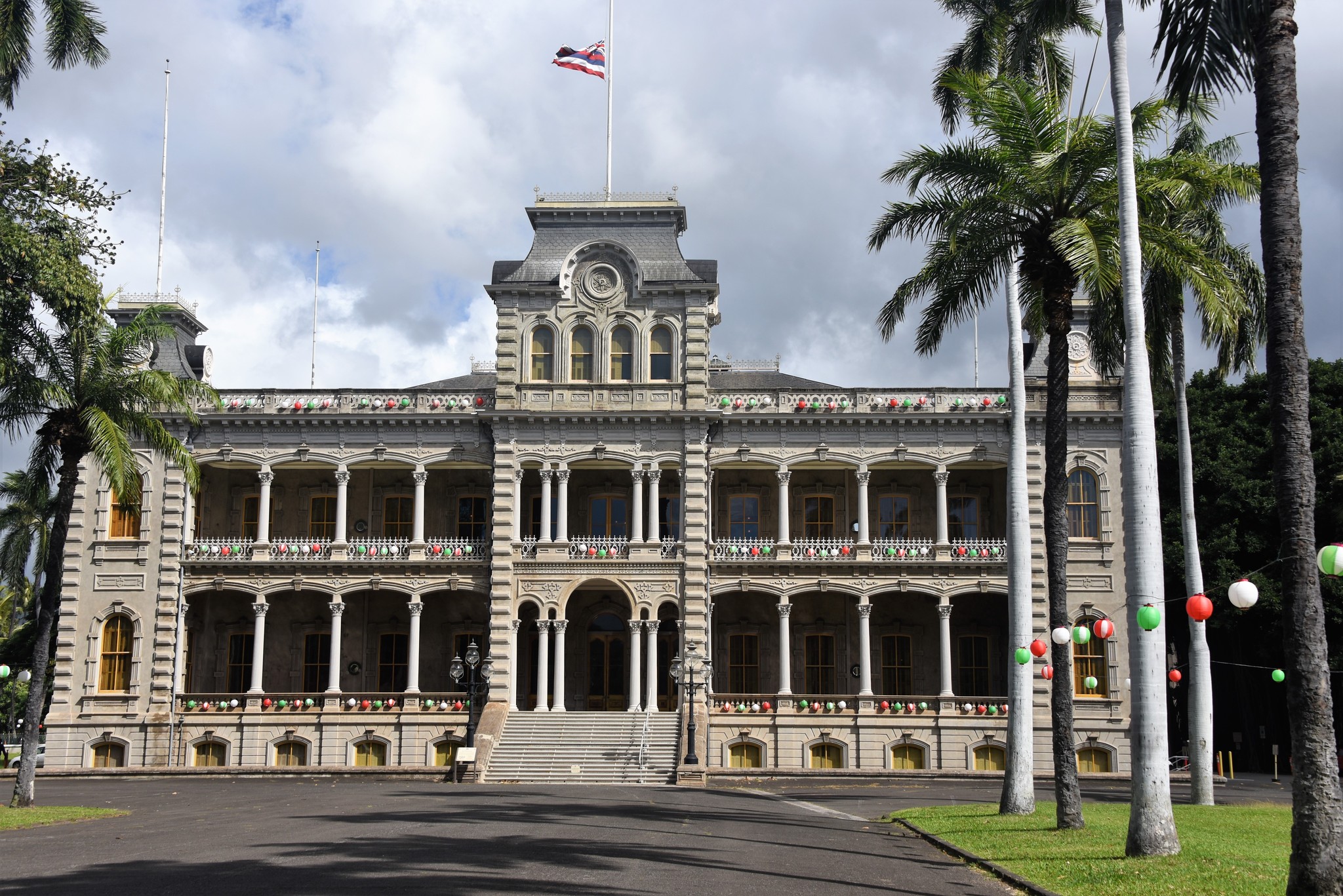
top-left (1186, 594), bottom-right (1213, 623)
top-left (1093, 618), bottom-right (1114, 639)
top-left (0, 664), bottom-right (10, 679)
top-left (729, 542), bottom-right (1000, 561)
top-left (1084, 676), bottom-right (1098, 690)
top-left (18, 669), bottom-right (31, 683)
top-left (17, 724), bottom-right (20, 728)
top-left (18, 719), bottom-right (23, 724)
top-left (1041, 665), bottom-right (1053, 680)
top-left (1030, 639), bottom-right (1046, 658)
top-left (1169, 669), bottom-right (1181, 682)
top-left (219, 398), bottom-right (484, 409)
top-left (1318, 543), bottom-right (1343, 579)
top-left (721, 396), bottom-right (1008, 409)
top-left (1015, 647), bottom-right (1030, 665)
top-left (39, 724), bottom-right (43, 728)
top-left (1052, 626), bottom-right (1070, 645)
top-left (1071, 624), bottom-right (1090, 645)
top-left (187, 698), bottom-right (470, 711)
top-left (722, 695), bottom-right (1009, 720)
top-left (1228, 579), bottom-right (1259, 611)
top-left (1137, 605), bottom-right (1161, 631)
top-left (200, 543), bottom-right (473, 556)
top-left (1272, 669), bottom-right (1285, 683)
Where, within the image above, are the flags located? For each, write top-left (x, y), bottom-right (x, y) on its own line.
top-left (552, 37), bottom-right (605, 80)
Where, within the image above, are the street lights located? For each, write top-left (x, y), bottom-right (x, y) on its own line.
top-left (450, 638), bottom-right (496, 764)
top-left (669, 637), bottom-right (713, 764)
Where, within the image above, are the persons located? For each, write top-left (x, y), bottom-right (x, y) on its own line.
top-left (0, 738), bottom-right (7, 761)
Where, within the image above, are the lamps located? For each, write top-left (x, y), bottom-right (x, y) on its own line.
top-left (721, 396), bottom-right (1006, 409)
top-left (579, 543), bottom-right (617, 558)
top-left (17, 724), bottom-right (20, 728)
top-left (39, 724), bottom-right (42, 728)
top-left (216, 397), bottom-right (483, 410)
top-left (1014, 539), bottom-right (1342, 691)
top-left (18, 669), bottom-right (31, 683)
top-left (730, 545), bottom-right (999, 558)
top-left (199, 542), bottom-right (472, 557)
top-left (723, 700), bottom-right (1009, 714)
top-left (0, 664), bottom-right (10, 678)
top-left (18, 718), bottom-right (23, 724)
top-left (187, 694), bottom-right (471, 710)
